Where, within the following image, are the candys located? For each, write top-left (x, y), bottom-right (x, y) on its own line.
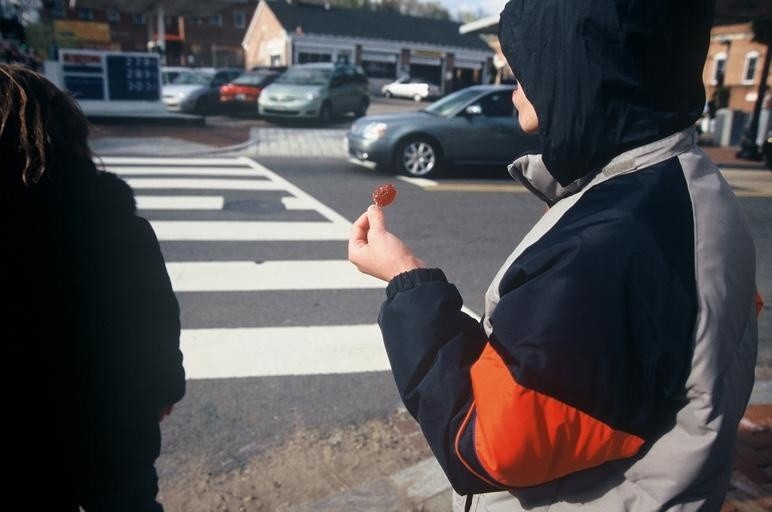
top-left (372, 184), bottom-right (397, 208)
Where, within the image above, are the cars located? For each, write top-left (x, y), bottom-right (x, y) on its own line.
top-left (156, 62), bottom-right (371, 124)
top-left (343, 84), bottom-right (542, 181)
top-left (380, 75), bottom-right (443, 101)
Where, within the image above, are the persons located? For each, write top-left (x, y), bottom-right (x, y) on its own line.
top-left (347, 2), bottom-right (765, 512)
top-left (2, 63), bottom-right (190, 510)
